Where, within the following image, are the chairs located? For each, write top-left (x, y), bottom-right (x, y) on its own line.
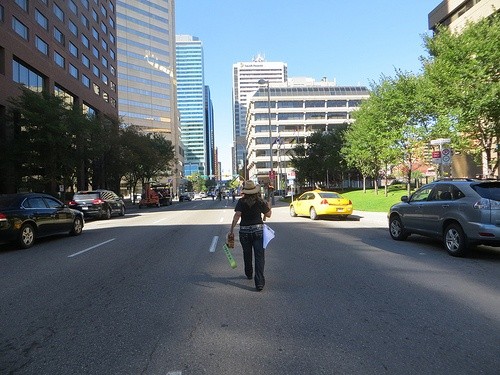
top-left (440, 192), bottom-right (452, 200)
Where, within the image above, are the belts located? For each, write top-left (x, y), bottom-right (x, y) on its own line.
top-left (241, 224), bottom-right (262, 229)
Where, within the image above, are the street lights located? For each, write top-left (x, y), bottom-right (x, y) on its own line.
top-left (234, 141), bottom-right (246, 185)
top-left (257, 80), bottom-right (275, 206)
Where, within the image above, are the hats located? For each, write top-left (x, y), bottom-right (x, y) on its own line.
top-left (241, 180), bottom-right (258, 194)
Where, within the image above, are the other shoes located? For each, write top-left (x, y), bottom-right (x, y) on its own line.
top-left (246, 274), bottom-right (252, 279)
top-left (256, 284), bottom-right (263, 290)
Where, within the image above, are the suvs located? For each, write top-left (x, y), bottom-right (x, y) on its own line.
top-left (388, 177), bottom-right (500, 257)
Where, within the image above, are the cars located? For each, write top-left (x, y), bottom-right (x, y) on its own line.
top-left (199, 192), bottom-right (207, 198)
top-left (289, 190), bottom-right (354, 220)
top-left (0, 193), bottom-right (85, 249)
top-left (70, 190), bottom-right (127, 220)
top-left (194, 194), bottom-right (203, 200)
top-left (179, 193), bottom-right (193, 202)
top-left (208, 192), bottom-right (216, 197)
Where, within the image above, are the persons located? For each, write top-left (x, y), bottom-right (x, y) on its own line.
top-left (232, 191), bottom-right (235, 202)
top-left (227, 192), bottom-right (229, 199)
top-left (230, 180), bottom-right (271, 291)
